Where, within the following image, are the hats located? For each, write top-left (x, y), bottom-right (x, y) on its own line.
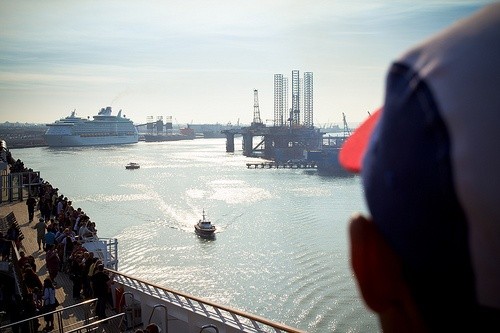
top-left (65, 197), bottom-right (68, 200)
top-left (338, 0), bottom-right (500, 333)
top-left (56, 188), bottom-right (59, 190)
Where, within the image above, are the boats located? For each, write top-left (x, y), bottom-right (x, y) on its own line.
top-left (307, 111), bottom-right (356, 177)
top-left (135, 114), bottom-right (195, 143)
top-left (125, 161), bottom-right (140, 170)
top-left (194, 208), bottom-right (217, 238)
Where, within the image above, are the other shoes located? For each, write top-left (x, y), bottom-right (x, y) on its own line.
top-left (29, 219), bottom-right (33, 223)
top-left (52, 280), bottom-right (56, 283)
top-left (43, 324), bottom-right (54, 330)
top-left (73, 295), bottom-right (83, 300)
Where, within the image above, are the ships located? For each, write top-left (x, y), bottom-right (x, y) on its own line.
top-left (41, 105), bottom-right (139, 147)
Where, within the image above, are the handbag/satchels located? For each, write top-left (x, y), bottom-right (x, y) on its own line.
top-left (41, 305), bottom-right (52, 312)
top-left (55, 297), bottom-right (60, 307)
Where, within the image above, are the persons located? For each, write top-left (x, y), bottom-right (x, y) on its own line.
top-left (346, 3), bottom-right (500, 333)
top-left (0, 151), bottom-right (110, 328)
